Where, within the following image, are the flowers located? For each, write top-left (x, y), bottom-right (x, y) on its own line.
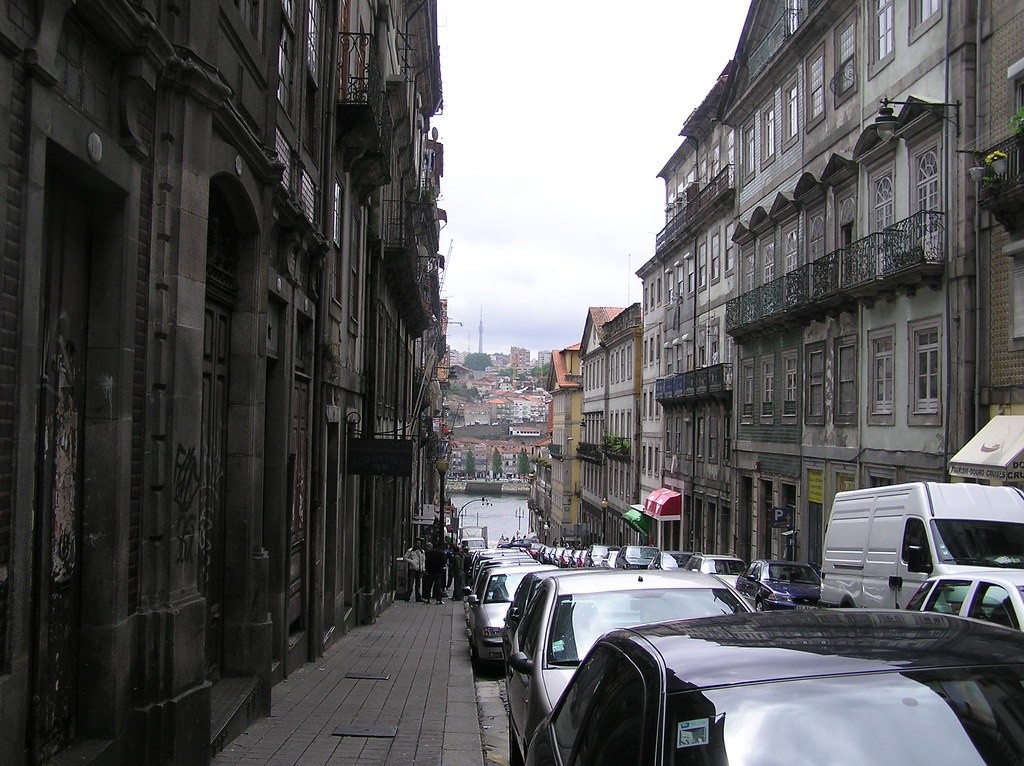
top-left (985, 151), bottom-right (1008, 165)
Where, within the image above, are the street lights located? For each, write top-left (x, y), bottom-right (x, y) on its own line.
top-left (434, 454), bottom-right (449, 598)
top-left (601, 497), bottom-right (609, 544)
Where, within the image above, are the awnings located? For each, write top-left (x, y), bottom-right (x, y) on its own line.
top-left (643, 487), bottom-right (681, 521)
top-left (948, 413), bottom-right (1024, 481)
top-left (621, 509), bottom-right (651, 538)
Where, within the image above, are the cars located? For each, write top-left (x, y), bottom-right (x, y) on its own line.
top-left (521, 606), bottom-right (1024, 765)
top-left (902, 570), bottom-right (1024, 633)
top-left (736, 558), bottom-right (821, 612)
top-left (459, 531), bottom-right (748, 766)
top-left (646, 550), bottom-right (697, 571)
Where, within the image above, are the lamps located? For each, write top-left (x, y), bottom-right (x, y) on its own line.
top-left (580, 418), bottom-right (586, 426)
top-left (875, 95), bottom-right (962, 142)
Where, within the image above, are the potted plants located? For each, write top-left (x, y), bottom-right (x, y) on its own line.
top-left (980, 176), bottom-right (1001, 199)
top-left (969, 147), bottom-right (986, 181)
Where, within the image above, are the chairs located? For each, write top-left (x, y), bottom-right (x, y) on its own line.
top-left (493, 582), bottom-right (509, 599)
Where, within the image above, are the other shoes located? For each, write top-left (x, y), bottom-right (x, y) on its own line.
top-left (442, 593), bottom-right (448, 597)
top-left (416, 599), bottom-right (425, 602)
top-left (405, 598), bottom-right (410, 602)
top-left (425, 599), bottom-right (431, 604)
top-left (436, 600), bottom-right (444, 604)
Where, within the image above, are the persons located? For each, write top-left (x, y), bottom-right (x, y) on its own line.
top-left (553, 538), bottom-right (589, 551)
top-left (404, 536), bottom-right (472, 605)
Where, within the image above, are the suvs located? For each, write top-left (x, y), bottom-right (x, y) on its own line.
top-left (614, 543), bottom-right (660, 571)
top-left (683, 552), bottom-right (748, 589)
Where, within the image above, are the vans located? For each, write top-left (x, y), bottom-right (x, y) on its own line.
top-left (815, 480), bottom-right (1024, 613)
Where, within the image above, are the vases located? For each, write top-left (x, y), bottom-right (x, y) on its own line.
top-left (992, 158), bottom-right (1008, 174)
